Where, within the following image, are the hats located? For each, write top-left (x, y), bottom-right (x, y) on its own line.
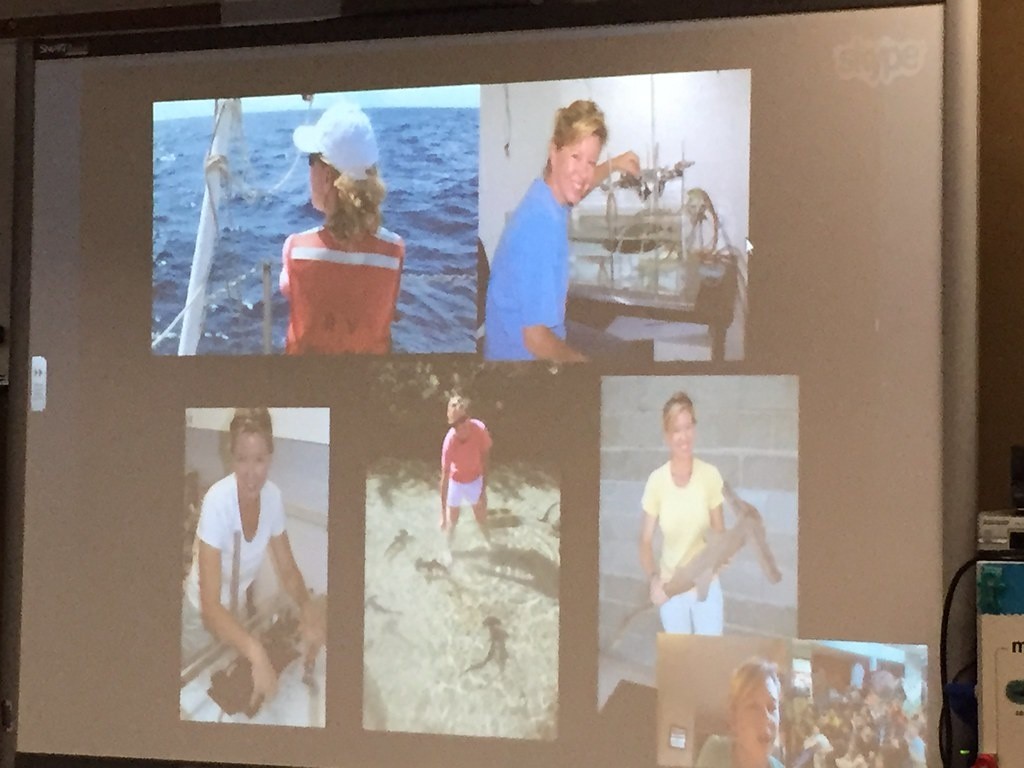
top-left (290, 102), bottom-right (380, 176)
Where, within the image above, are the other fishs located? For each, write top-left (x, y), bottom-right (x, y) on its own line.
top-left (363, 505), bottom-right (521, 674)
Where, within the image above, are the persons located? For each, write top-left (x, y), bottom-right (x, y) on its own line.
top-left (438, 392), bottom-right (501, 553)
top-left (181, 407), bottom-right (326, 728)
top-left (277, 100), bottom-right (409, 355)
top-left (639, 393), bottom-right (728, 635)
top-left (482, 99), bottom-right (646, 360)
top-left (696, 656), bottom-right (928, 768)
